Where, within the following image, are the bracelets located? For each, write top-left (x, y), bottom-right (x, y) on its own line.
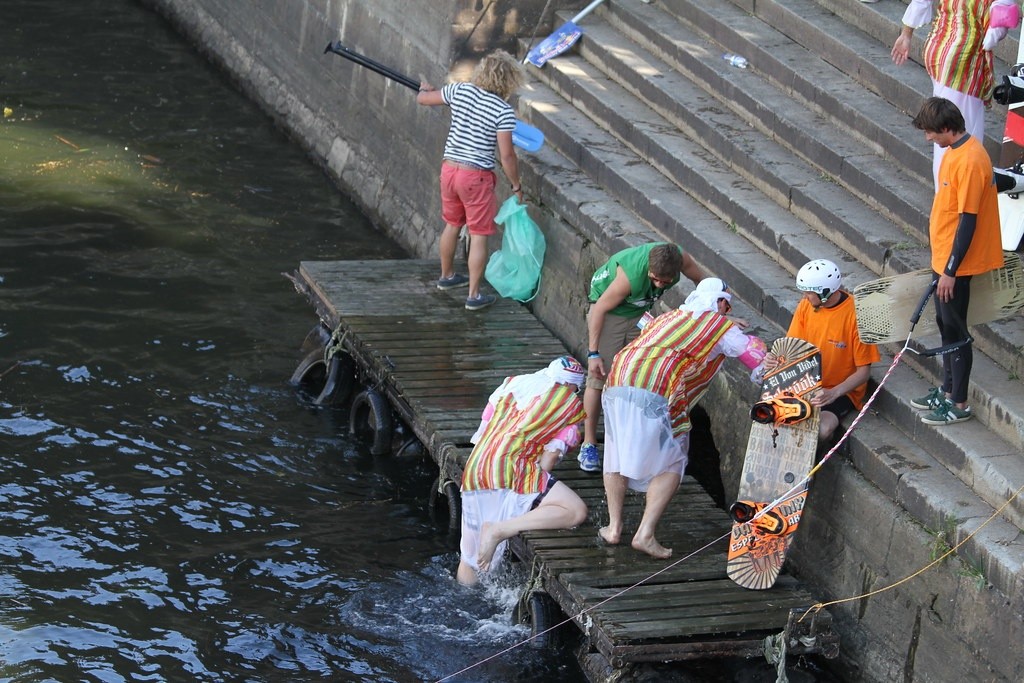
top-left (588, 351), bottom-right (599, 358)
top-left (419, 88), bottom-right (427, 91)
top-left (511, 184), bottom-right (520, 192)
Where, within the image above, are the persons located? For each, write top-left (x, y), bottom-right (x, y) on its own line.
top-left (455, 357), bottom-right (586, 584)
top-left (597, 279), bottom-right (767, 559)
top-left (891, 0), bottom-right (1019, 195)
top-left (577, 239), bottom-right (730, 472)
top-left (910, 97), bottom-right (1005, 427)
top-left (417, 50), bottom-right (524, 310)
top-left (764, 259), bottom-right (881, 442)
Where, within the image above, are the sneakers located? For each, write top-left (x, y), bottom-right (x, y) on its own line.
top-left (466, 293), bottom-right (496, 310)
top-left (921, 403), bottom-right (971, 425)
top-left (749, 391), bottom-right (812, 447)
top-left (577, 442), bottom-right (603, 472)
top-left (722, 500), bottom-right (787, 535)
top-left (436, 273), bottom-right (468, 290)
top-left (910, 385), bottom-right (953, 410)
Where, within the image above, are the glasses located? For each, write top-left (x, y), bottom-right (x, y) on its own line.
top-left (720, 298), bottom-right (732, 313)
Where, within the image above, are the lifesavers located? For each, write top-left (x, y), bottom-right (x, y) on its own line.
top-left (508, 590), bottom-right (563, 651)
top-left (426, 475), bottom-right (461, 532)
top-left (349, 390), bottom-right (394, 456)
top-left (288, 319), bottom-right (352, 408)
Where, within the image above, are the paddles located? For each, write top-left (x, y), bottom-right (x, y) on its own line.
top-left (323, 39), bottom-right (545, 152)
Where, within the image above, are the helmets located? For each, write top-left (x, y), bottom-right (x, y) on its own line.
top-left (796, 258), bottom-right (843, 304)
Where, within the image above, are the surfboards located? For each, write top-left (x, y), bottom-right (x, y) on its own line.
top-left (853, 248), bottom-right (1024, 346)
top-left (726, 337), bottom-right (822, 592)
